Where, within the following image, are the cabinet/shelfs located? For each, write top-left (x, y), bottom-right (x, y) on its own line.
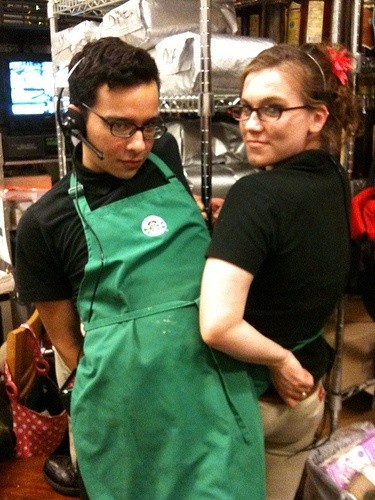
top-left (228, 0), bottom-right (375, 194)
top-left (50, 15), bottom-right (360, 433)
top-left (0, 0), bottom-right (74, 169)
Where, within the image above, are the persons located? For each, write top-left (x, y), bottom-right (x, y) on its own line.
top-left (14, 36), bottom-right (265, 500)
top-left (200, 43), bottom-right (351, 500)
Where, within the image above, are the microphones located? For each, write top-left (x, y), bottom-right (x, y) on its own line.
top-left (70, 128), bottom-right (104, 160)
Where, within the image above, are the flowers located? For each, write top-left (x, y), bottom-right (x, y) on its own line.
top-left (327, 46), bottom-right (353, 86)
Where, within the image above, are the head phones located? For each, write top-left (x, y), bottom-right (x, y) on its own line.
top-left (57, 46), bottom-right (87, 142)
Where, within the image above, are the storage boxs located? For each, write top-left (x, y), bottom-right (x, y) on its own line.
top-left (3, 132), bottom-right (46, 160)
top-left (301, 421), bottom-right (375, 500)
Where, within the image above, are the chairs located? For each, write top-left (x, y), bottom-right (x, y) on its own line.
top-left (0, 308), bottom-right (44, 458)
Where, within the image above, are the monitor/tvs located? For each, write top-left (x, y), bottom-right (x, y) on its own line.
top-left (8, 60), bottom-right (71, 116)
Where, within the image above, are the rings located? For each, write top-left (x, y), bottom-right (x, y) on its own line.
top-left (300, 391), bottom-right (307, 400)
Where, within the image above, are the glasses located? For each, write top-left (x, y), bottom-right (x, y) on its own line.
top-left (78, 101), bottom-right (168, 141)
top-left (234, 100), bottom-right (317, 124)
